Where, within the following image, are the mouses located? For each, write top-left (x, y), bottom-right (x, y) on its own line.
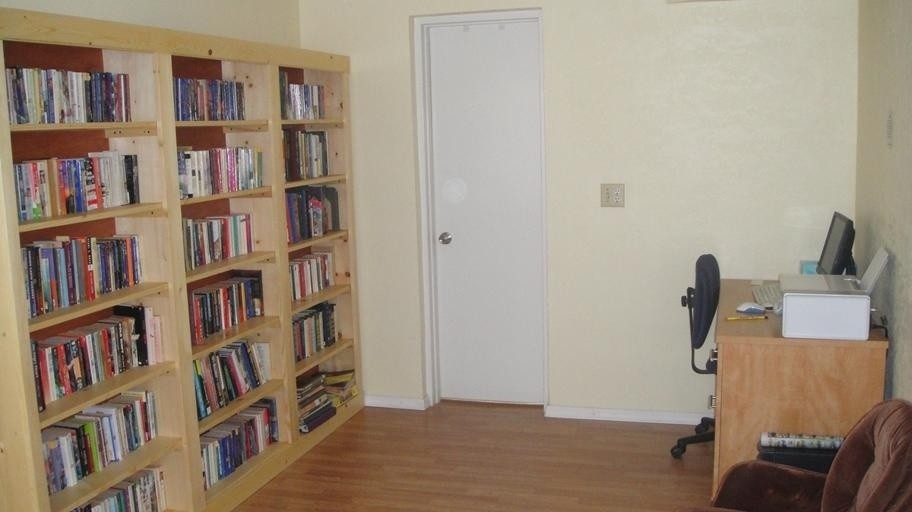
top-left (736, 301), bottom-right (766, 315)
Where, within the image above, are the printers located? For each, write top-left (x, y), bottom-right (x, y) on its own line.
top-left (778, 247), bottom-right (891, 340)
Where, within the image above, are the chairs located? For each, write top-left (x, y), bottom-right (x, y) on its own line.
top-left (713, 399), bottom-right (912, 511)
top-left (672, 254), bottom-right (720, 458)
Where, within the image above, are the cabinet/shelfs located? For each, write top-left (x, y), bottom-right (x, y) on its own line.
top-left (0, 9), bottom-right (365, 512)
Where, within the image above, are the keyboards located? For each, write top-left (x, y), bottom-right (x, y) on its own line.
top-left (750, 282), bottom-right (784, 306)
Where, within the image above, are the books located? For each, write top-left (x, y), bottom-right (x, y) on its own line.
top-left (41, 389), bottom-right (157, 494)
top-left (200, 398), bottom-right (279, 491)
top-left (279, 71), bottom-right (325, 119)
top-left (297, 370), bottom-right (358, 433)
top-left (282, 129), bottom-right (328, 181)
top-left (6, 69), bottom-right (131, 125)
top-left (21, 235), bottom-right (144, 318)
top-left (285, 186), bottom-right (339, 243)
top-left (173, 77), bottom-right (245, 121)
top-left (292, 303), bottom-right (336, 362)
top-left (31, 304), bottom-right (162, 412)
top-left (289, 251), bottom-right (335, 300)
top-left (187, 269), bottom-right (264, 342)
top-left (74, 466), bottom-right (167, 512)
top-left (192, 338), bottom-right (271, 418)
top-left (177, 146), bottom-right (262, 200)
top-left (183, 214), bottom-right (253, 269)
top-left (14, 151), bottom-right (140, 223)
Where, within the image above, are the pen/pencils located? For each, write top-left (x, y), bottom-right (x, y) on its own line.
top-left (727, 315), bottom-right (767, 321)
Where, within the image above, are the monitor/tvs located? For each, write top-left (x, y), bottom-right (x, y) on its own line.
top-left (816, 211), bottom-right (857, 274)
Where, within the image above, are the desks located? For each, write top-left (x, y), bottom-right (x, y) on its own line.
top-left (713, 277), bottom-right (886, 508)
